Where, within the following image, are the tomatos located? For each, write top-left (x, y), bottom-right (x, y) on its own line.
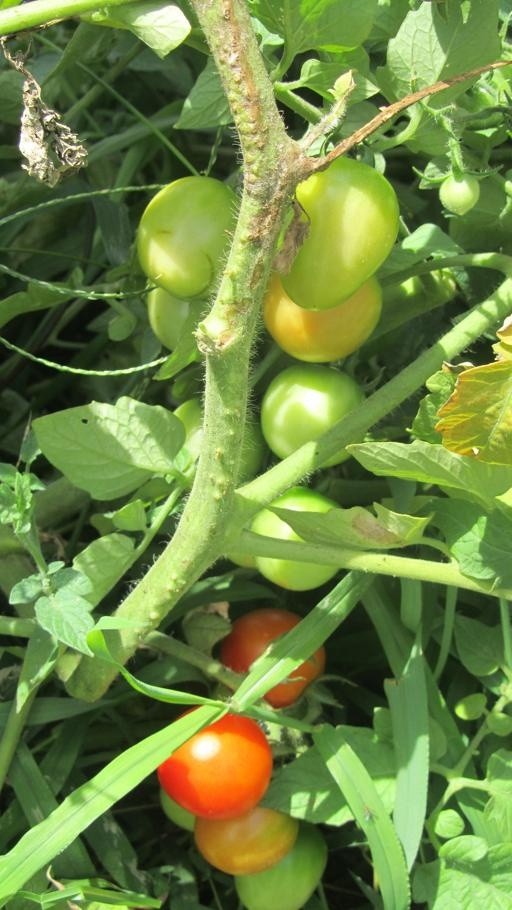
top-left (133, 157), bottom-right (399, 910)
top-left (439, 173), bottom-right (480, 216)
